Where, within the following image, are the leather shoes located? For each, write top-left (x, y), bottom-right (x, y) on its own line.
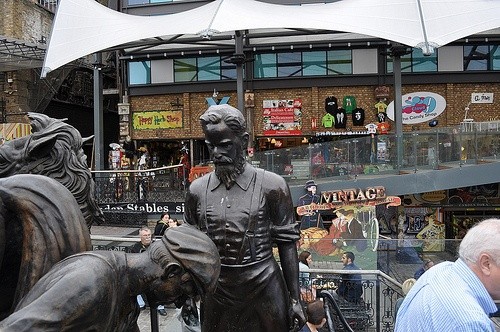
top-left (158, 309), bottom-right (167, 316)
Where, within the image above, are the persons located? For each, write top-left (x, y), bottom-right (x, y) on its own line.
top-left (0, 224), bottom-right (221, 332)
top-left (394, 218), bottom-right (500, 332)
top-left (330, 251), bottom-right (363, 302)
top-left (299, 301), bottom-right (326, 332)
top-left (181, 104), bottom-right (305, 332)
top-left (298, 251), bottom-right (323, 292)
top-left (394, 278), bottom-right (417, 322)
top-left (414, 259), bottom-right (436, 279)
top-left (154, 213), bottom-right (181, 238)
top-left (129, 227), bottom-right (168, 316)
